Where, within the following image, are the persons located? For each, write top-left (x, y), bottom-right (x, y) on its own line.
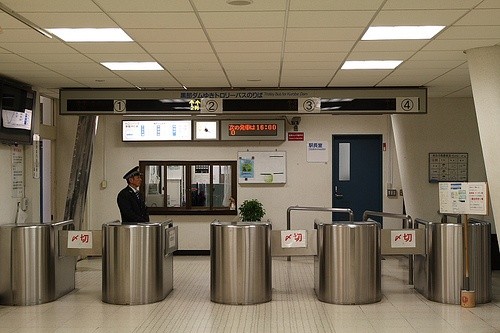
top-left (117, 166), bottom-right (150, 224)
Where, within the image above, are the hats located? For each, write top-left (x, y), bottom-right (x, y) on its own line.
top-left (123, 166), bottom-right (145, 179)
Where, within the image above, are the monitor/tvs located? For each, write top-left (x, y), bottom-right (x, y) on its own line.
top-left (0, 83), bottom-right (37, 146)
top-left (121, 120), bottom-right (192, 142)
